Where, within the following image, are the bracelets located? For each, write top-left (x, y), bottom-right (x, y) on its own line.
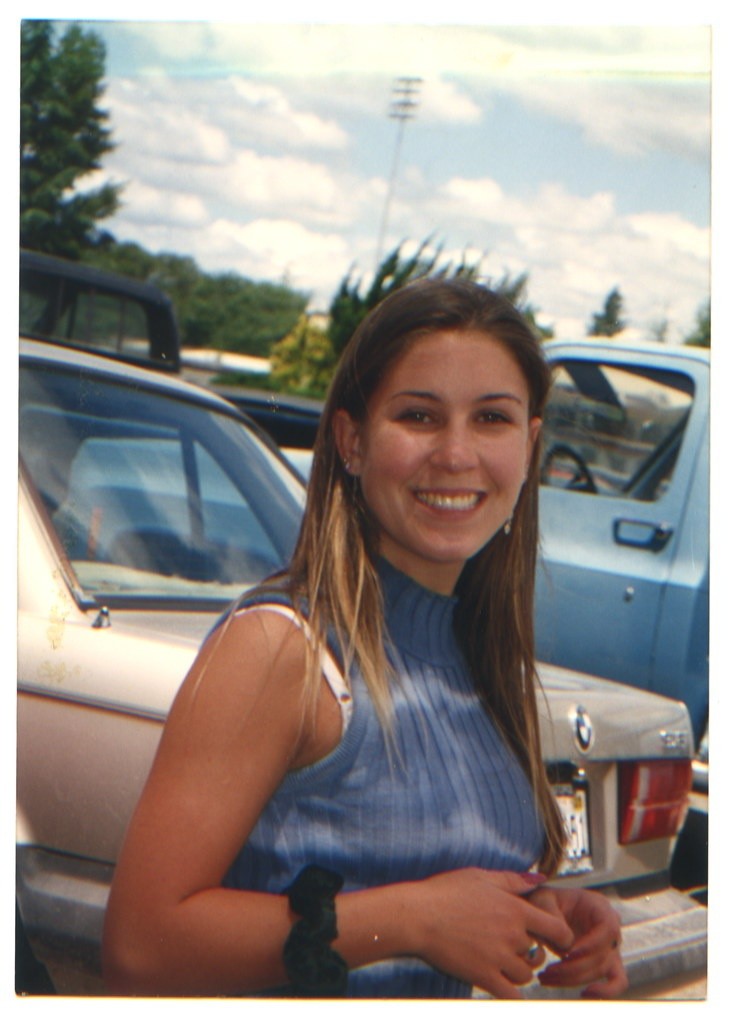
top-left (283, 864), bottom-right (349, 997)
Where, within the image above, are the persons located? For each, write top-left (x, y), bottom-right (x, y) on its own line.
top-left (102, 278), bottom-right (628, 998)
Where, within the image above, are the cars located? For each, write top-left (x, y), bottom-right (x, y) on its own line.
top-left (16, 334), bottom-right (708, 1003)
top-left (19, 247), bottom-right (322, 441)
top-left (50, 336), bottom-right (710, 790)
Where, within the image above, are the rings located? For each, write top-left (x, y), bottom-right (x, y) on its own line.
top-left (613, 939), bottom-right (617, 947)
top-left (529, 944), bottom-right (538, 956)
